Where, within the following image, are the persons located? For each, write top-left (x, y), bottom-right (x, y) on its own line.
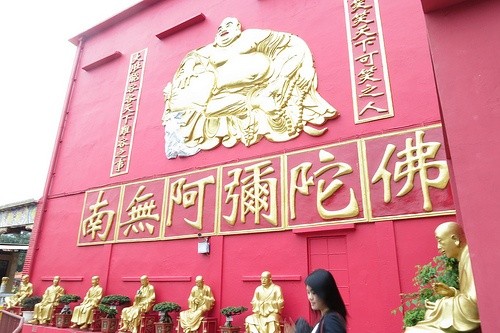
top-left (28, 276), bottom-right (66, 325)
top-left (5, 275), bottom-right (33, 311)
top-left (284, 269), bottom-right (348, 333)
top-left (404, 221), bottom-right (480, 333)
top-left (180, 276), bottom-right (215, 333)
top-left (244, 271), bottom-right (284, 333)
top-left (118, 275), bottom-right (155, 333)
top-left (70, 275), bottom-right (103, 330)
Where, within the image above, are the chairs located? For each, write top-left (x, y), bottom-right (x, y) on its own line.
top-left (120, 300), bottom-right (158, 333)
top-left (3, 303), bottom-right (24, 315)
top-left (87, 308), bottom-right (106, 331)
top-left (177, 312), bottom-right (218, 333)
top-left (47, 303), bottom-right (64, 326)
top-left (121, 302), bottom-right (159, 333)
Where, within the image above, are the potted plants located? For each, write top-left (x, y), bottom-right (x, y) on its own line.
top-left (219, 305), bottom-right (248, 333)
top-left (98, 294), bottom-right (130, 333)
top-left (21, 295), bottom-right (43, 324)
top-left (152, 301), bottom-right (181, 333)
top-left (55, 294), bottom-right (81, 328)
